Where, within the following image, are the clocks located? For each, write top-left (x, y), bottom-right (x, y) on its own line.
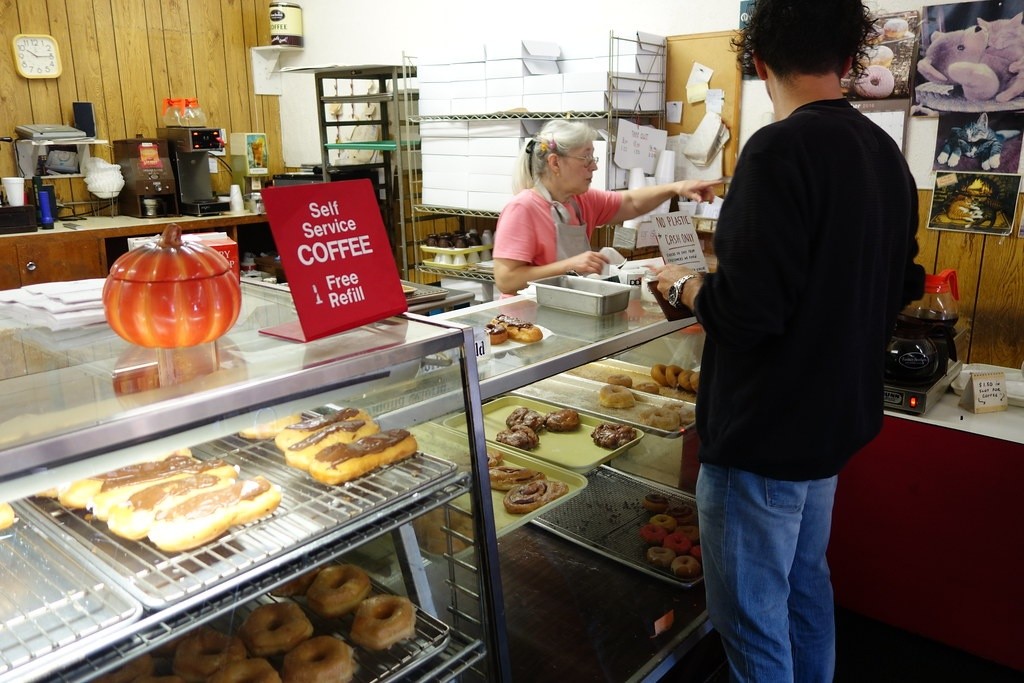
top-left (13, 34), bottom-right (61, 78)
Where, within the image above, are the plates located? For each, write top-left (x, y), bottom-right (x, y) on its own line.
top-left (1005, 380), bottom-right (1024, 408)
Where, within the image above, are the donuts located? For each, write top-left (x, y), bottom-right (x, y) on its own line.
top-left (485, 313), bottom-right (702, 579)
top-left (98, 559), bottom-right (416, 683)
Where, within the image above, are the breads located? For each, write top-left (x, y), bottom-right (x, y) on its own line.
top-left (0, 407), bottom-right (418, 552)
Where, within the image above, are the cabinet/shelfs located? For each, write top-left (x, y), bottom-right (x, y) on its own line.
top-left (415, 26), bottom-right (674, 283)
top-left (0, 273), bottom-right (515, 682)
top-left (0, 238), bottom-right (111, 381)
top-left (318, 61), bottom-right (462, 283)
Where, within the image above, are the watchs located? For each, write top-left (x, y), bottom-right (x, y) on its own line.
top-left (667, 273), bottom-right (701, 308)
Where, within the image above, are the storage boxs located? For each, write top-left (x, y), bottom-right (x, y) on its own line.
top-left (418, 36), bottom-right (673, 208)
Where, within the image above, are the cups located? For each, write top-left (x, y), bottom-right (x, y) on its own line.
top-left (622, 150), bottom-right (697, 233)
top-left (1, 177), bottom-right (25, 207)
top-left (428, 229), bottom-right (493, 266)
top-left (229, 185), bottom-right (245, 215)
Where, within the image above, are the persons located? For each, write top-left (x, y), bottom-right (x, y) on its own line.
top-left (655, 0), bottom-right (924, 682)
top-left (491, 119), bottom-right (724, 322)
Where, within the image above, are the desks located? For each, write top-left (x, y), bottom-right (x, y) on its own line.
top-left (0, 212), bottom-right (267, 383)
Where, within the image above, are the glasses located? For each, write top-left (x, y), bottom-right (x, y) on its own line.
top-left (546, 153), bottom-right (598, 168)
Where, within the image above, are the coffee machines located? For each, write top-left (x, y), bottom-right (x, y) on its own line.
top-left (154, 126), bottom-right (236, 217)
top-left (112, 135), bottom-right (181, 217)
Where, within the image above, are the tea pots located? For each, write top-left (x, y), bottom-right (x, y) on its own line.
top-left (894, 267), bottom-right (960, 338)
top-left (178, 98), bottom-right (208, 127)
top-left (161, 96), bottom-right (185, 127)
